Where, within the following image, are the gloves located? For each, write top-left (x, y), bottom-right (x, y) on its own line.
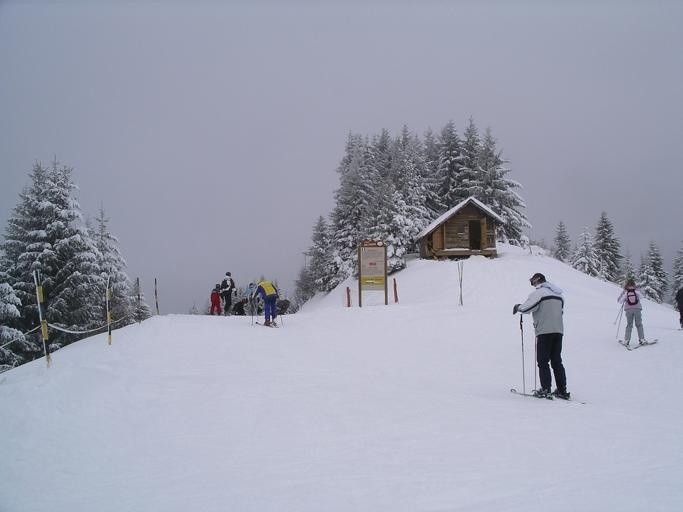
top-left (513, 304), bottom-right (521, 314)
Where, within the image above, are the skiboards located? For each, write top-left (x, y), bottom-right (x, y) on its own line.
top-left (510, 389), bottom-right (587, 405)
top-left (255, 321), bottom-right (280, 329)
top-left (619, 339), bottom-right (657, 351)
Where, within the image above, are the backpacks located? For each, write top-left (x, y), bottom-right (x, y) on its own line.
top-left (220, 278), bottom-right (229, 289)
top-left (626, 288), bottom-right (639, 305)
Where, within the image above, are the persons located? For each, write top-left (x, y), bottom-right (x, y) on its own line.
top-left (674, 286), bottom-right (682, 328)
top-left (241, 282), bottom-right (259, 315)
top-left (512, 272), bottom-right (566, 398)
top-left (231, 298), bottom-right (248, 316)
top-left (616, 279), bottom-right (647, 346)
top-left (250, 276), bottom-right (279, 327)
top-left (208, 283), bottom-right (222, 316)
top-left (219, 271), bottom-right (237, 316)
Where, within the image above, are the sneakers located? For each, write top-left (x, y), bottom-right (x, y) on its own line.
top-left (639, 338), bottom-right (647, 344)
top-left (534, 388), bottom-right (551, 397)
top-left (264, 320), bottom-right (270, 326)
top-left (553, 386), bottom-right (567, 396)
top-left (624, 340), bottom-right (629, 345)
